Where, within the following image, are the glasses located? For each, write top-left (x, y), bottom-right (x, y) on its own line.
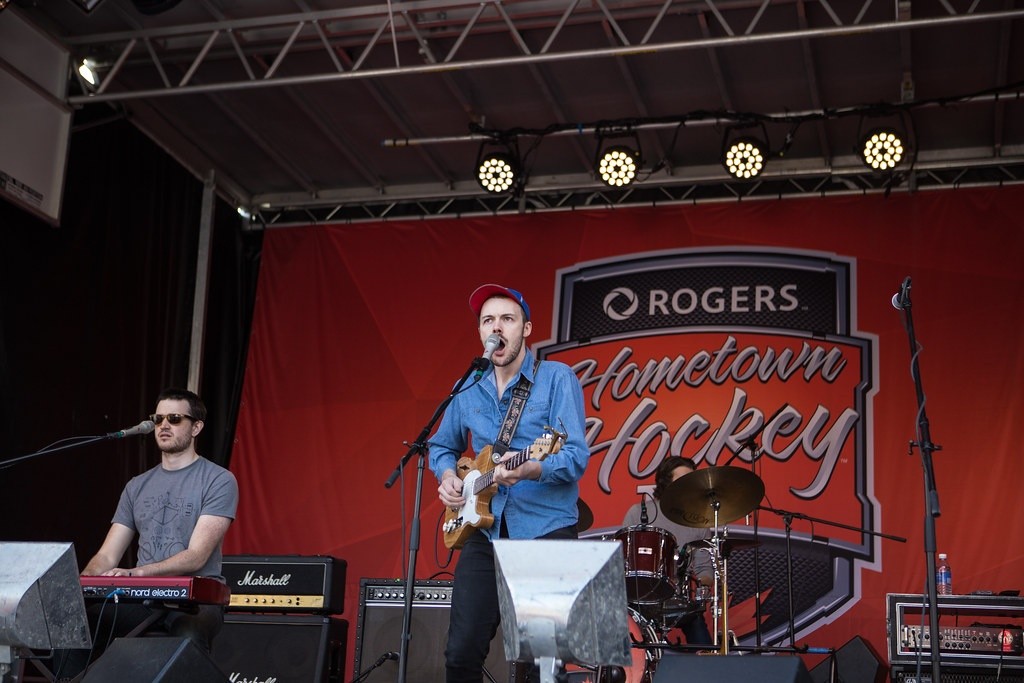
top-left (150, 413), bottom-right (196, 425)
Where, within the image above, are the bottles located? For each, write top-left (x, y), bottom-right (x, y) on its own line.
top-left (936, 553), bottom-right (952, 595)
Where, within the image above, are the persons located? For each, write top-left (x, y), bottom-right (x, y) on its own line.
top-left (429, 284), bottom-right (591, 683)
top-left (53, 390), bottom-right (239, 679)
top-left (622, 455), bottom-right (717, 653)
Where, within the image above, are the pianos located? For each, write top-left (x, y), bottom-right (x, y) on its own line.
top-left (21, 574), bottom-right (233, 683)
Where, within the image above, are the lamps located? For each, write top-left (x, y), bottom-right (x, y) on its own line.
top-left (857, 102), bottom-right (911, 172)
top-left (724, 120), bottom-right (772, 181)
top-left (594, 140), bottom-right (642, 189)
top-left (472, 125), bottom-right (529, 196)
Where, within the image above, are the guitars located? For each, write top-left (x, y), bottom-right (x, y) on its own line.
top-left (442, 415), bottom-right (570, 551)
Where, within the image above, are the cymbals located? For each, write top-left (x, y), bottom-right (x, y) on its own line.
top-left (682, 537), bottom-right (766, 550)
top-left (575, 496), bottom-right (595, 533)
top-left (659, 464), bottom-right (766, 528)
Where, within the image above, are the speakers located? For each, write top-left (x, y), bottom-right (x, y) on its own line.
top-left (890, 665), bottom-right (1024, 683)
top-left (81, 613), bottom-right (349, 683)
top-left (652, 636), bottom-right (883, 683)
top-left (354, 577), bottom-right (517, 683)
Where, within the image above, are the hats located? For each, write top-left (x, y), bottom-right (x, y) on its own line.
top-left (469, 284), bottom-right (529, 322)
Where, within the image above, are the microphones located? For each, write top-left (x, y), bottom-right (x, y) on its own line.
top-left (641, 499), bottom-right (648, 523)
top-left (608, 665), bottom-right (625, 683)
top-left (677, 544), bottom-right (687, 565)
top-left (473, 334), bottom-right (501, 382)
top-left (891, 276), bottom-right (913, 310)
top-left (384, 652), bottom-right (401, 662)
top-left (114, 421), bottom-right (156, 438)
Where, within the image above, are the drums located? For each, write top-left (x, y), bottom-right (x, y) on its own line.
top-left (614, 524), bottom-right (679, 605)
top-left (633, 550), bottom-right (692, 627)
